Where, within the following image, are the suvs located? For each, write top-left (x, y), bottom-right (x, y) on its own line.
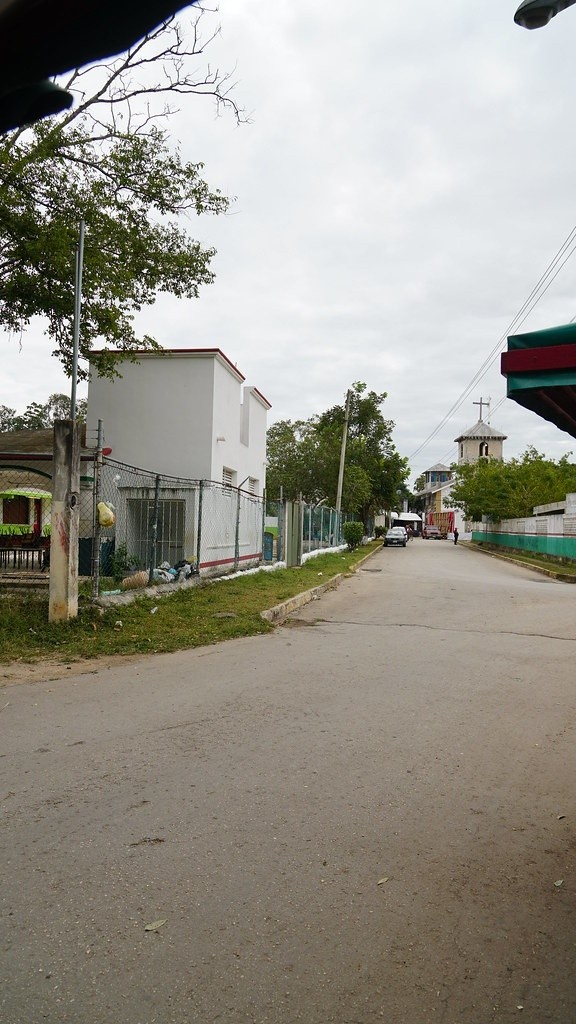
top-left (394, 524), bottom-right (409, 541)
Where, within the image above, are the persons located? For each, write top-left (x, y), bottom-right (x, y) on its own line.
top-left (407, 527), bottom-right (412, 540)
top-left (454, 528), bottom-right (459, 545)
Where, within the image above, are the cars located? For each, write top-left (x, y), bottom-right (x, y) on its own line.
top-left (383, 526), bottom-right (407, 549)
top-left (422, 524), bottom-right (443, 540)
